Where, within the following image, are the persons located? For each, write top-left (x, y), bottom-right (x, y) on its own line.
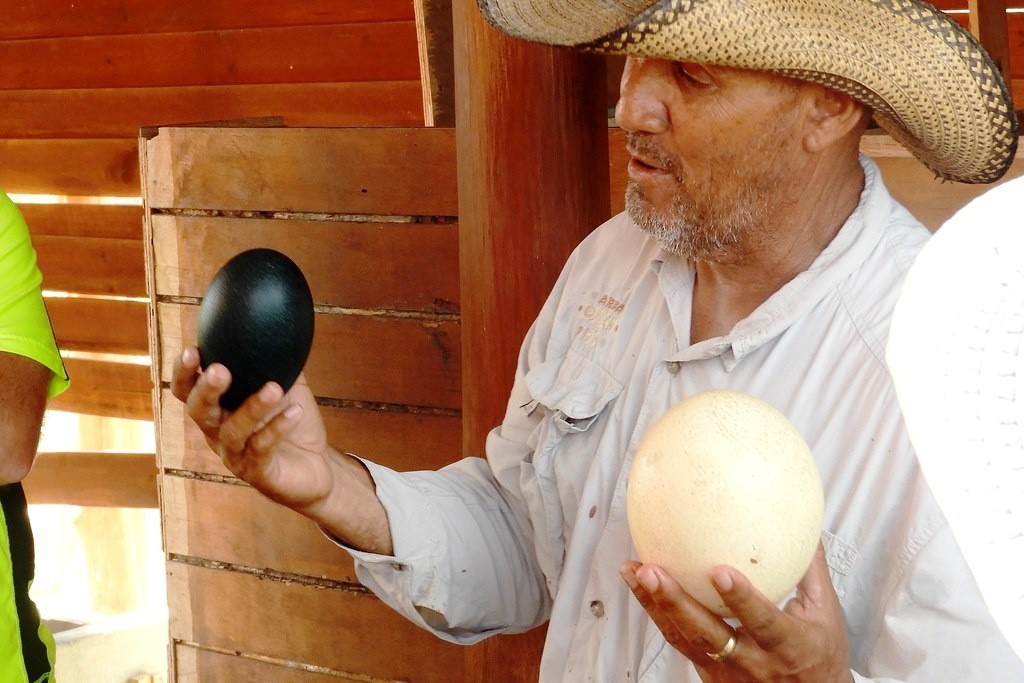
top-left (173, 1), bottom-right (1024, 683)
top-left (0, 190), bottom-right (71, 683)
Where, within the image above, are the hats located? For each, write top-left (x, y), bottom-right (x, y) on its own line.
top-left (477, 0), bottom-right (1021, 185)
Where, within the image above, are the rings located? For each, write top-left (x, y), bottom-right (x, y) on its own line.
top-left (706, 626), bottom-right (738, 661)
